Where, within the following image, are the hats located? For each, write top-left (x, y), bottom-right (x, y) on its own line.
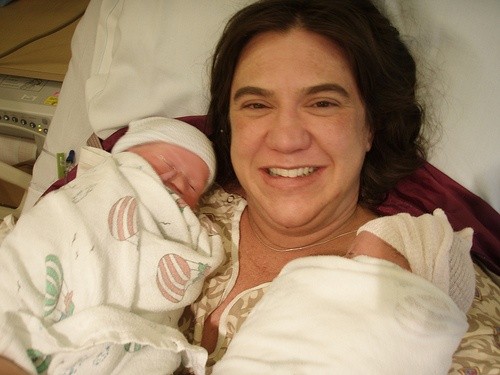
top-left (110, 117), bottom-right (217, 192)
top-left (357, 207), bottom-right (479, 315)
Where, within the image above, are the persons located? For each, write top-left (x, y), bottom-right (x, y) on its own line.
top-left (1, 0), bottom-right (430, 374)
top-left (1, 115), bottom-right (226, 375)
top-left (205, 207), bottom-right (476, 375)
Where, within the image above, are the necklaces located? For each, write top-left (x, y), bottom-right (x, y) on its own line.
top-left (244, 202), bottom-right (363, 253)
